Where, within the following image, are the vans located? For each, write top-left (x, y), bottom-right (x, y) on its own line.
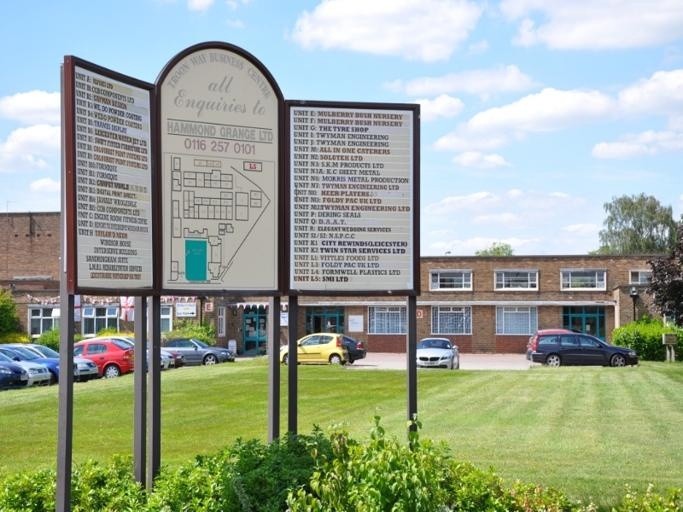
top-left (529, 332), bottom-right (638, 367)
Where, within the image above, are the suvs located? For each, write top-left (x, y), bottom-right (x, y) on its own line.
top-left (526, 328), bottom-right (580, 361)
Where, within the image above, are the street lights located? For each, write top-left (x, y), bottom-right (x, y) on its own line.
top-left (629, 286), bottom-right (638, 321)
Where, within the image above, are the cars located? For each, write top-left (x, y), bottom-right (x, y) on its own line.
top-left (158, 337), bottom-right (237, 368)
top-left (0, 335), bottom-right (185, 389)
top-left (415, 336), bottom-right (462, 370)
top-left (276, 332), bottom-right (350, 368)
top-left (303, 336), bottom-right (367, 365)
